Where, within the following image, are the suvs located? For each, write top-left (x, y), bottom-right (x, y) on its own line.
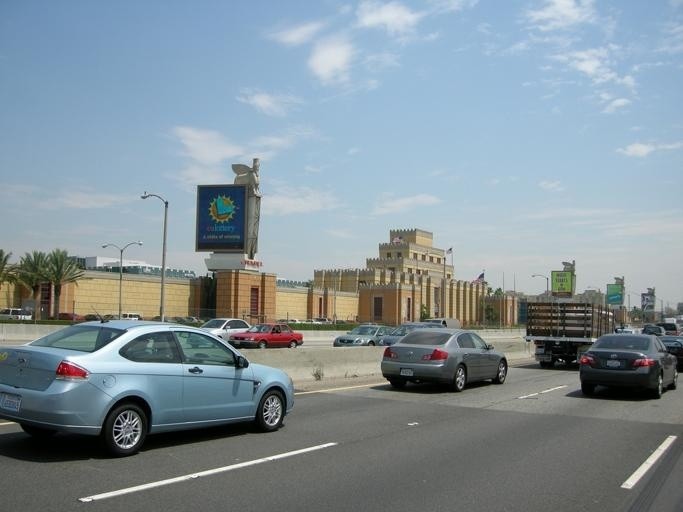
top-left (0, 308), bottom-right (31, 321)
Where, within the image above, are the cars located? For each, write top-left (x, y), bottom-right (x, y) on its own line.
top-left (576, 323), bottom-right (683, 400)
top-left (0, 321), bottom-right (295, 457)
top-left (228, 323), bottom-right (304, 349)
top-left (380, 329), bottom-right (509, 391)
top-left (49, 313), bottom-right (331, 324)
top-left (331, 322), bottom-right (448, 346)
top-left (186, 318), bottom-right (258, 349)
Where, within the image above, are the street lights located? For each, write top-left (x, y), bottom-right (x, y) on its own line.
top-left (139, 190), bottom-right (169, 324)
top-left (100, 240), bottom-right (142, 320)
